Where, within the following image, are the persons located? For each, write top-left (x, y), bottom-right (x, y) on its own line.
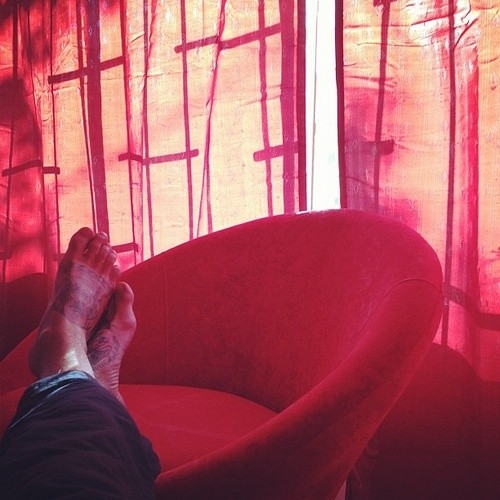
top-left (1, 226), bottom-right (162, 500)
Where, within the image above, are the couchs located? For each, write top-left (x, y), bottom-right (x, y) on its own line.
top-left (0, 207), bottom-right (447, 499)
